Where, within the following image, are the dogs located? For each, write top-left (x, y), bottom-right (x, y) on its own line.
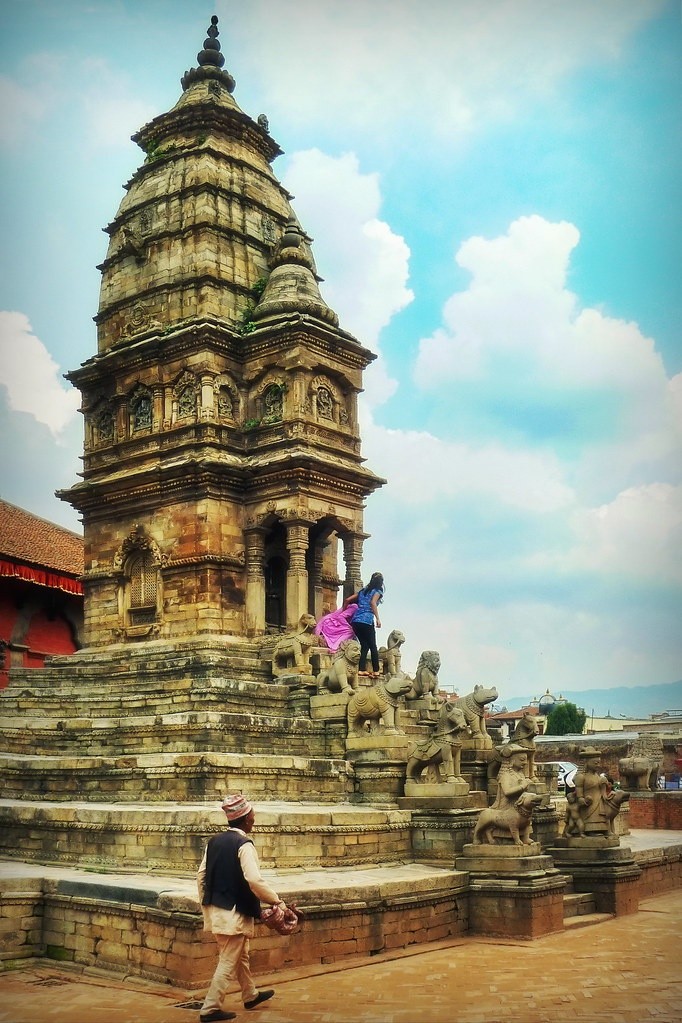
top-left (565, 790), bottom-right (630, 837)
top-left (436, 685), bottom-right (498, 739)
top-left (473, 792), bottom-right (543, 846)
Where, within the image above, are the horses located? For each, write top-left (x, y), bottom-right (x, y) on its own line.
top-left (405, 702), bottom-right (468, 784)
top-left (487, 710), bottom-right (540, 783)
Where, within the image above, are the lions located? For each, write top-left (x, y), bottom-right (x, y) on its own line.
top-left (406, 650), bottom-right (444, 704)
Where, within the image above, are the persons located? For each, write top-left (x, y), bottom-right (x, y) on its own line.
top-left (196, 794), bottom-right (291, 1023)
top-left (343, 571), bottom-right (384, 678)
top-left (566, 745), bottom-right (608, 838)
top-left (494, 745), bottom-right (536, 810)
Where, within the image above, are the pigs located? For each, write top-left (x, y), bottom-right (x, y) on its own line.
top-left (347, 678), bottom-right (413, 738)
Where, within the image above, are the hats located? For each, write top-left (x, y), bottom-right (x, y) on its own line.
top-left (221, 794), bottom-right (251, 820)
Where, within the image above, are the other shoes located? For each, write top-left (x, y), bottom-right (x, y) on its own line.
top-left (373, 672), bottom-right (380, 677)
top-left (244, 989), bottom-right (274, 1009)
top-left (358, 670), bottom-right (373, 676)
top-left (200, 1009), bottom-right (236, 1022)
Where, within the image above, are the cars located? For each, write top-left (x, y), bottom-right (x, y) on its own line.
top-left (556, 762), bottom-right (579, 789)
